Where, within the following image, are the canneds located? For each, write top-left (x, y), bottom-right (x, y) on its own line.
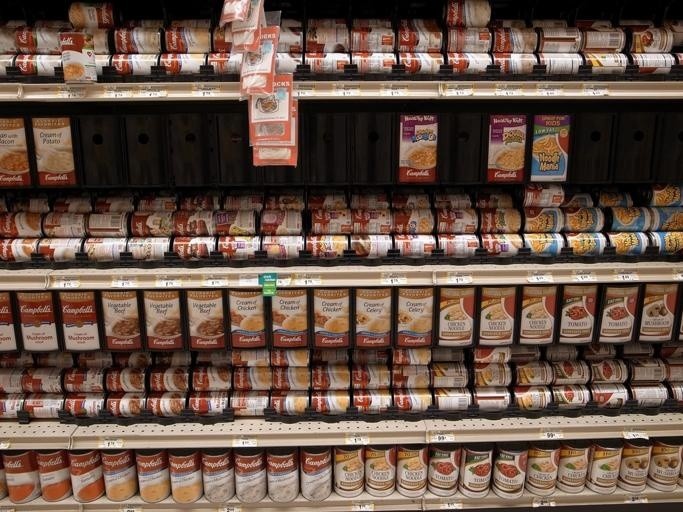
top-left (561, 193), bottom-right (593, 208)
top-left (0, 55), bottom-right (16, 78)
top-left (66, 450), bottom-right (104, 503)
top-left (628, 383), bottom-right (668, 409)
top-left (594, 191), bottom-right (634, 207)
top-left (626, 357), bottom-right (666, 383)
top-left (265, 195), bottom-right (302, 210)
top-left (583, 344), bottom-right (616, 360)
top-left (365, 447), bottom-right (396, 496)
top-left (649, 207), bottom-right (683, 231)
top-left (352, 390), bottom-right (393, 416)
top-left (605, 207), bottom-right (650, 232)
top-left (352, 365), bottom-right (390, 389)
top-left (310, 391), bottom-right (350, 415)
top-left (233, 450), bottom-right (267, 502)
top-left (552, 361), bottom-right (590, 385)
top-left (229, 391), bottom-right (269, 417)
top-left (477, 207), bottom-right (521, 234)
top-left (522, 208), bottom-right (564, 233)
top-left (305, 27), bottom-right (350, 52)
top-left (170, 19), bottom-right (212, 28)
top-left (3, 451), bottom-right (40, 503)
top-left (298, 448), bottom-right (332, 501)
top-left (43, 212), bottom-right (88, 238)
top-left (473, 364), bottom-right (511, 387)
top-left (491, 19), bottom-right (526, 28)
top-left (0, 367), bottom-right (24, 394)
top-left (668, 381), bottom-right (683, 403)
top-left (400, 18), bottom-right (437, 28)
top-left (639, 184), bottom-right (683, 206)
top-left (94, 29), bottom-right (115, 55)
top-left (95, 55), bottom-right (111, 76)
top-left (538, 53), bottom-right (583, 75)
top-left (352, 52), bottom-right (397, 74)
top-left (429, 444), bottom-right (460, 496)
top-left (306, 235), bottom-right (348, 257)
top-left (664, 20), bottom-right (683, 47)
top-left (35, 21), bottom-right (72, 30)
top-left (218, 236), bottom-right (261, 260)
top-left (579, 26), bottom-right (626, 52)
top-left (154, 351), bottom-right (192, 369)
top-left (70, 2), bottom-right (118, 27)
top-left (625, 27), bottom-right (673, 53)
top-left (167, 449), bottom-right (203, 503)
top-left (524, 443), bottom-right (559, 495)
top-left (105, 367), bottom-right (147, 393)
top-left (517, 183), bottom-right (564, 207)
top-left (54, 197), bottom-right (92, 213)
top-left (231, 348), bottom-right (271, 367)
top-left (397, 28), bottom-right (442, 53)
top-left (192, 366), bottom-right (233, 392)
top-left (510, 345), bottom-right (540, 362)
top-left (473, 192), bottom-right (513, 208)
top-left (112, 54), bottom-right (160, 75)
top-left (262, 236), bottom-right (304, 259)
top-left (173, 237), bottom-right (217, 260)
top-left (113, 26), bottom-right (163, 52)
top-left (562, 207), bottom-right (604, 232)
top-left (352, 348), bottom-right (389, 366)
top-left (213, 209), bottom-right (258, 236)
top-left (0, 238), bottom-right (40, 262)
top-left (394, 234), bottom-right (436, 257)
top-left (664, 358), bottom-right (683, 381)
top-left (630, 53), bottom-right (676, 74)
top-left (0, 351), bottom-right (34, 367)
top-left (214, 27), bottom-right (234, 52)
top-left (312, 349), bottom-right (349, 365)
top-left (5, 21), bottom-right (27, 29)
top-left (473, 387), bottom-right (510, 411)
top-left (492, 27), bottom-right (537, 52)
top-left (434, 389), bottom-right (471, 410)
top-left (350, 27), bottom-right (395, 52)
top-left (445, 27), bottom-right (492, 52)
top-left (172, 210), bottom-right (216, 236)
top-left (160, 53), bottom-right (206, 74)
top-left (472, 346), bottom-right (510, 364)
top-left (105, 393), bottom-right (148, 419)
top-left (397, 446), bottom-right (429, 497)
top-left (10, 197), bottom-right (49, 214)
top-left (0, 26), bottom-right (37, 54)
top-left (101, 451), bottom-right (138, 502)
top-left (351, 209), bottom-right (392, 234)
top-left (35, 450), bottom-right (71, 501)
top-left (589, 384), bottom-right (628, 409)
top-left (77, 350), bottom-right (113, 368)
top-left (460, 445), bottom-right (492, 498)
top-left (532, 19), bottom-right (567, 28)
top-left (480, 234), bottom-right (523, 257)
top-left (94, 196), bottom-right (134, 213)
top-left (393, 389), bottom-right (432, 413)
top-left (393, 208), bottom-right (433, 234)
top-left (86, 211), bottom-right (129, 237)
top-left (622, 342), bottom-right (653, 359)
top-left (583, 52), bottom-right (628, 73)
top-left (188, 391), bottom-right (228, 416)
top-left (272, 367), bottom-right (310, 390)
top-left (493, 53), bottom-right (538, 74)
top-left (617, 439), bottom-right (654, 492)
top-left (443, 0), bottom-right (491, 27)
top-left (277, 27), bottom-right (303, 52)
top-left (392, 194), bottom-right (429, 209)
top-left (223, 194), bottom-right (264, 212)
top-left (195, 349), bottom-right (231, 367)
top-left (308, 19), bottom-right (347, 28)
top-left (83, 237), bottom-right (126, 263)
top-left (265, 449), bottom-right (299, 503)
top-left (350, 234), bottom-right (392, 258)
top-left (180, 196), bottom-right (220, 210)
top-left (575, 20), bottom-right (612, 29)
top-left (545, 345), bottom-right (577, 361)
top-left (0, 212), bottom-right (44, 238)
top-left (432, 347), bottom-right (464, 363)
top-left (24, 393), bottom-right (65, 419)
top-left (588, 359), bottom-right (629, 383)
top-left (149, 367), bottom-right (190, 393)
top-left (522, 233), bottom-right (564, 257)
top-left (124, 20), bottom-right (166, 29)
top-left (269, 390), bottom-right (310, 416)
top-left (430, 363), bottom-right (468, 388)
top-left (138, 196), bottom-right (177, 212)
top-left (648, 232), bottom-right (683, 257)
top-left (398, 52), bottom-right (444, 74)
top-left (114, 350), bottom-right (153, 368)
top-left (493, 444), bottom-right (528, 499)
top-left (311, 209), bottom-right (352, 235)
top-left (131, 211), bottom-right (173, 236)
top-left (392, 347), bottom-right (431, 365)
top-left (557, 441), bottom-right (590, 493)
top-left (392, 364), bottom-right (430, 388)
top-left (234, 367), bottom-right (273, 390)
top-left (38, 238), bottom-right (84, 262)
top-left (208, 53), bottom-right (243, 75)
top-left (586, 440), bottom-right (623, 494)
top-left (512, 361), bottom-right (552, 385)
top-left (536, 27), bottom-right (581, 52)
top-left (550, 386), bottom-right (590, 409)
top-left (308, 193), bottom-right (347, 210)
top-left (0, 394), bottom-right (27, 420)
top-left (165, 28), bottom-right (211, 53)
top-left (353, 19), bottom-right (391, 28)
top-left (435, 208), bottom-right (478, 234)
top-left (618, 20), bottom-right (655, 29)
top-left (136, 451), bottom-right (170, 502)
top-left (271, 347), bottom-right (310, 368)
top-left (22, 367), bottom-right (65, 393)
top-left (35, 27), bottom-right (61, 53)
top-left (660, 341), bottom-right (683, 359)
top-left (513, 386), bottom-right (552, 410)
top-left (35, 352), bottom-right (75, 367)
top-left (434, 195), bottom-right (471, 209)
top-left (16, 54), bottom-right (62, 77)
top-left (334, 446), bottom-right (364, 497)
top-left (312, 365), bottom-right (350, 390)
top-left (648, 440), bottom-right (683, 491)
top-left (438, 234), bottom-right (479, 257)
top-left (202, 451), bottom-right (235, 503)
top-left (127, 237), bottom-right (170, 260)
top-left (563, 232), bottom-right (605, 257)
top-left (147, 392), bottom-right (187, 418)
top-left (275, 53), bottom-right (303, 74)
top-left (446, 53), bottom-right (492, 74)
top-left (65, 367), bottom-right (107, 391)
top-left (305, 53), bottom-right (350, 74)
top-left (64, 392), bottom-right (105, 418)
top-left (351, 194), bottom-right (388, 210)
top-left (260, 209), bottom-right (302, 235)
top-left (605, 232), bottom-right (648, 256)
